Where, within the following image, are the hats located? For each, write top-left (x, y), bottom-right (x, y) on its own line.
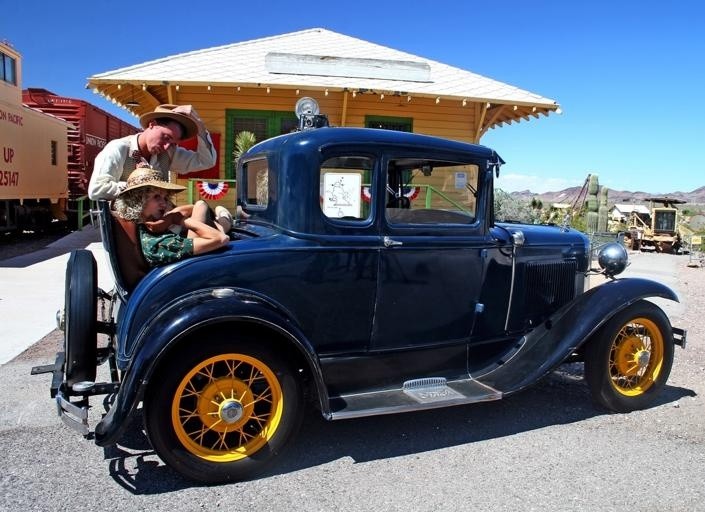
top-left (119, 168), bottom-right (188, 195)
top-left (139, 103), bottom-right (200, 141)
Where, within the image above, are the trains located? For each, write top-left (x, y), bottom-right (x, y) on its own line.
top-left (0, 33), bottom-right (143, 239)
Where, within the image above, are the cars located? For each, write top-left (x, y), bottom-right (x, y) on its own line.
top-left (30, 120), bottom-right (690, 490)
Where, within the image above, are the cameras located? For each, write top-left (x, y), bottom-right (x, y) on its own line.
top-left (300, 114), bottom-right (328, 128)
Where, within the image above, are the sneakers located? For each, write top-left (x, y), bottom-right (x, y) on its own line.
top-left (214, 205), bottom-right (233, 235)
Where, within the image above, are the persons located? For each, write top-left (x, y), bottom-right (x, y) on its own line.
top-left (88, 104), bottom-right (231, 266)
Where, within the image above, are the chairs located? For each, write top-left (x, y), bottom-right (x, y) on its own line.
top-left (103, 207), bottom-right (152, 296)
